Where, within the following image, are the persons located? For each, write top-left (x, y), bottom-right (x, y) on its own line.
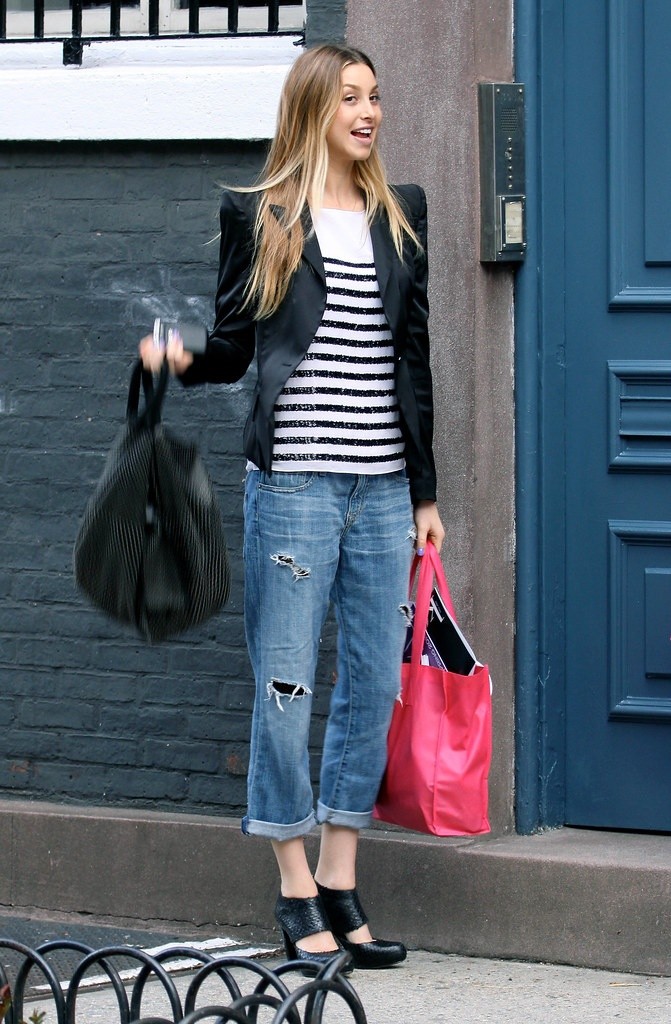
top-left (139, 44), bottom-right (447, 977)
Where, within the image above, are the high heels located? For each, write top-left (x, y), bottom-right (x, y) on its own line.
top-left (275, 888), bottom-right (353, 977)
top-left (312, 876), bottom-right (406, 969)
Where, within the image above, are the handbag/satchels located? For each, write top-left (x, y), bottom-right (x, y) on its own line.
top-left (72, 353), bottom-right (230, 641)
top-left (372, 539), bottom-right (492, 836)
